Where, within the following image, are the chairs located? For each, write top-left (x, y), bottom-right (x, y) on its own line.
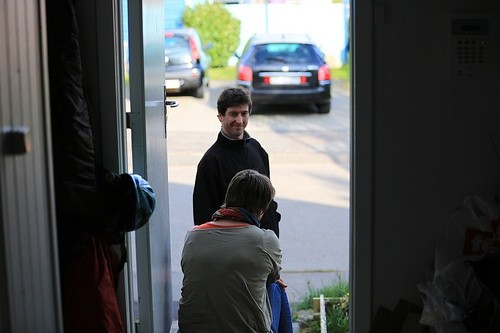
top-left (296, 47), bottom-right (309, 59)
top-left (255, 49), bottom-right (272, 60)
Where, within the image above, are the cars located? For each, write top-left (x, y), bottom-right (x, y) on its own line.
top-left (164, 27), bottom-right (213, 98)
top-left (232, 34), bottom-right (331, 114)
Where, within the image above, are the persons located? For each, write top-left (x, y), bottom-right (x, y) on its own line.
top-left (193, 87), bottom-right (281, 240)
top-left (176, 170), bottom-right (294, 333)
